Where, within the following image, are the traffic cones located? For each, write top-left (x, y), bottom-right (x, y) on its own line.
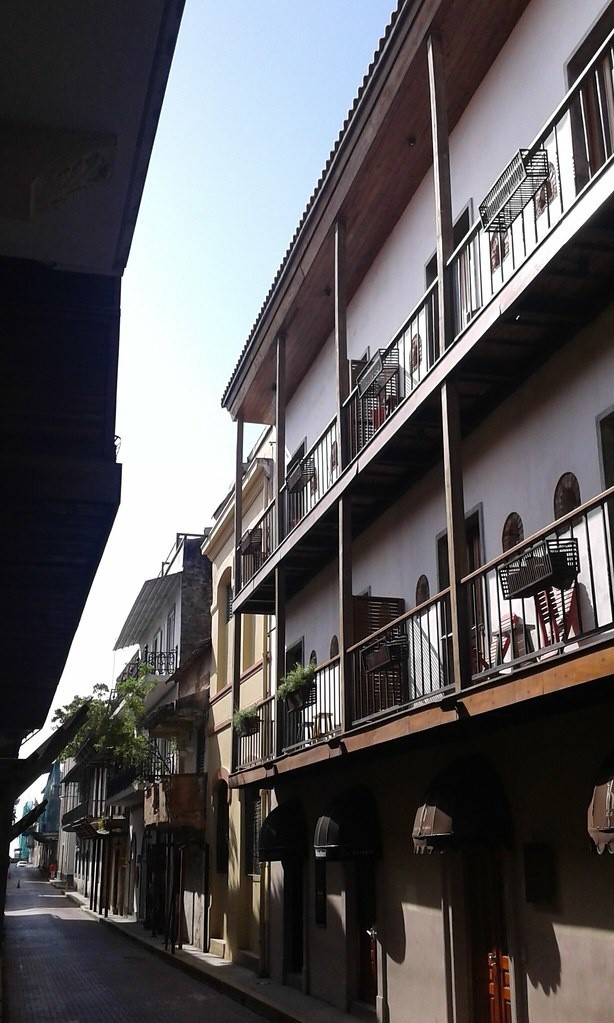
top-left (16, 880), bottom-right (21, 888)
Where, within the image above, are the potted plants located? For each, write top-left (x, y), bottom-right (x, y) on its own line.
top-left (172, 737), bottom-right (195, 756)
top-left (231, 703), bottom-right (257, 731)
top-left (91, 814), bottom-right (125, 831)
top-left (274, 657), bottom-right (315, 700)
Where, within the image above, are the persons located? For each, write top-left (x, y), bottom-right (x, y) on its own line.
top-left (50, 862), bottom-right (57, 879)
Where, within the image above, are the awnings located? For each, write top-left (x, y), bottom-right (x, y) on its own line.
top-left (412, 753), bottom-right (515, 853)
top-left (113, 571), bottom-right (181, 651)
top-left (257, 798), bottom-right (311, 860)
top-left (586, 750), bottom-right (614, 855)
top-left (313, 785), bottom-right (384, 860)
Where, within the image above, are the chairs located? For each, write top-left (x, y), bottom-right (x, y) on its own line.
top-left (473, 576), bottom-right (576, 681)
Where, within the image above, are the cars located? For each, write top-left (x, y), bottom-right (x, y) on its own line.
top-left (17, 861), bottom-right (28, 869)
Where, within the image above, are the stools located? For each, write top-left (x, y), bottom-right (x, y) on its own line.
top-left (296, 712), bottom-right (333, 749)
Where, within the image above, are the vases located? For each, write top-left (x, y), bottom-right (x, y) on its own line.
top-left (366, 644), bottom-right (401, 670)
top-left (506, 552), bottom-right (567, 594)
